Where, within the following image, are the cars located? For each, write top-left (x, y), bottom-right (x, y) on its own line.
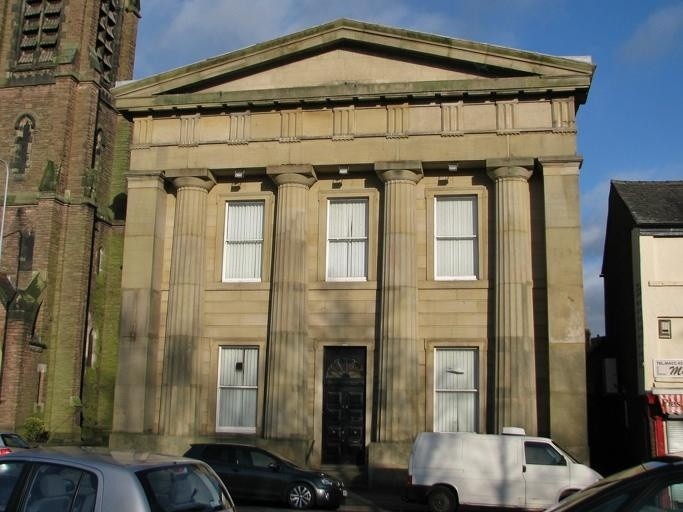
top-left (181, 442), bottom-right (348, 510)
top-left (544, 457), bottom-right (682, 511)
top-left (0, 446), bottom-right (239, 512)
top-left (0, 433), bottom-right (34, 456)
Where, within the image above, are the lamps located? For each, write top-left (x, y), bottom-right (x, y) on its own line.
top-left (448, 164), bottom-right (458, 171)
top-left (234, 169), bottom-right (245, 178)
top-left (339, 165), bottom-right (349, 174)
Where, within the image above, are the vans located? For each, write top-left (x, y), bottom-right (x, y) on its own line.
top-left (407, 426), bottom-right (605, 510)
